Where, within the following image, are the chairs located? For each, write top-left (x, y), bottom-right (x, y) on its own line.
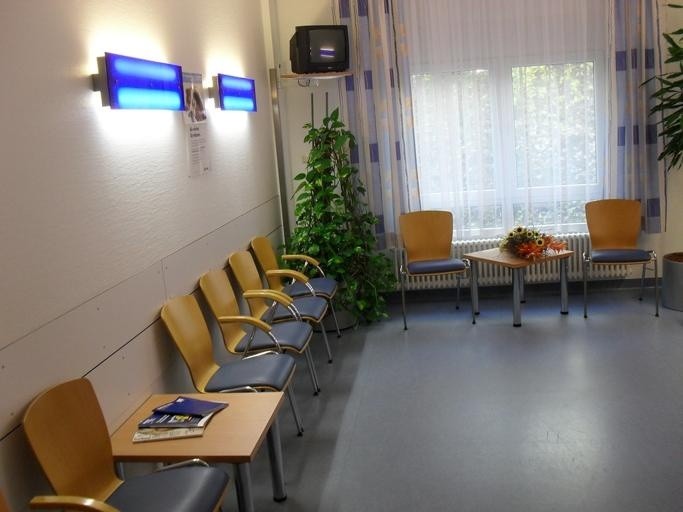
top-left (396, 208), bottom-right (476, 331)
top-left (582, 199), bottom-right (659, 318)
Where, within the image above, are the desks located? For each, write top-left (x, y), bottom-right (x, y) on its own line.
top-left (463, 245), bottom-right (573, 327)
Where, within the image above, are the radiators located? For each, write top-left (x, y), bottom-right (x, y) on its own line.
top-left (382, 232), bottom-right (631, 290)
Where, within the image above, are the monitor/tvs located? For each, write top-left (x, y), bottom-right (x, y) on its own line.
top-left (289, 24), bottom-right (351, 75)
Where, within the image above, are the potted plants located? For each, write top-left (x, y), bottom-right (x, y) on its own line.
top-left (275, 107), bottom-right (395, 331)
top-left (637, 20), bottom-right (682, 313)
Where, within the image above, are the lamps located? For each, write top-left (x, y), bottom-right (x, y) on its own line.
top-left (206, 73), bottom-right (259, 114)
top-left (89, 51), bottom-right (189, 113)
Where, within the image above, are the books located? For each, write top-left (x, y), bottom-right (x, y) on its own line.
top-left (132, 394), bottom-right (229, 444)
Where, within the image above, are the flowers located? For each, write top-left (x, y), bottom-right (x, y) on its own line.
top-left (499, 226), bottom-right (556, 258)
